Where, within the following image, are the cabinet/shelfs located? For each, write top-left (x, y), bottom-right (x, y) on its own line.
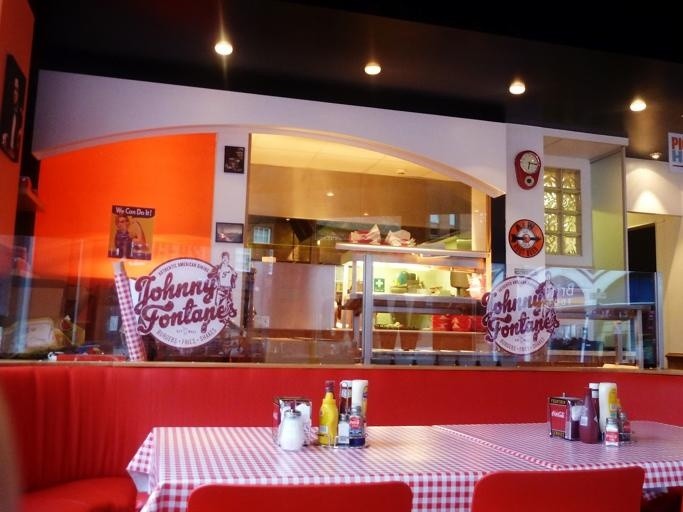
top-left (544, 301), bottom-right (656, 367)
top-left (335, 242), bottom-right (499, 365)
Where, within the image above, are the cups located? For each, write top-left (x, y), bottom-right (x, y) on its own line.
top-left (341, 309), bottom-right (353, 329)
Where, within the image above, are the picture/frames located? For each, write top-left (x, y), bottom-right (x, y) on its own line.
top-left (215, 222), bottom-right (244, 243)
top-left (224, 146), bottom-right (244, 173)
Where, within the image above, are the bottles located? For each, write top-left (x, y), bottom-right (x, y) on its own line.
top-left (579, 382), bottom-right (631, 446)
top-left (272, 381), bottom-right (364, 451)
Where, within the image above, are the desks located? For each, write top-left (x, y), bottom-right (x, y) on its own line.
top-left (125, 419), bottom-right (683, 512)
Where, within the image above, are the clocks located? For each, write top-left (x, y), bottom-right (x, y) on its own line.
top-left (515, 150), bottom-right (541, 189)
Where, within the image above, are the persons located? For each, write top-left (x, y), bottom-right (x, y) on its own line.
top-left (113, 213), bottom-right (146, 258)
top-left (207, 252), bottom-right (237, 306)
top-left (534, 270), bottom-right (557, 320)
